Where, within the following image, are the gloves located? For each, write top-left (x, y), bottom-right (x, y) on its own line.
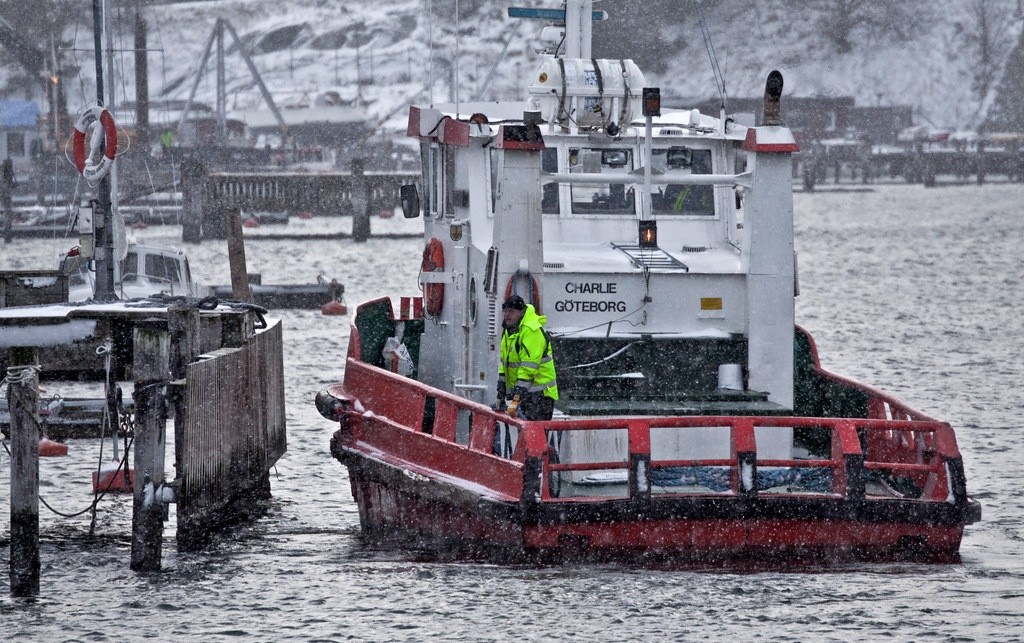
top-left (507, 401), bottom-right (519, 417)
top-left (491, 399), bottom-right (508, 412)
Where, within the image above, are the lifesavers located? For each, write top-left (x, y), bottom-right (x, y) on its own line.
top-left (69, 106), bottom-right (119, 180)
top-left (416, 236), bottom-right (444, 318)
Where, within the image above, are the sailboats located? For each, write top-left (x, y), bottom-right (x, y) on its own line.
top-left (56, 1), bottom-right (198, 302)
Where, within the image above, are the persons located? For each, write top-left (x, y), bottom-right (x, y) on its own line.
top-left (495, 295), bottom-right (559, 440)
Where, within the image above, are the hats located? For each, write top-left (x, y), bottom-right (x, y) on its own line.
top-left (501, 294), bottom-right (528, 310)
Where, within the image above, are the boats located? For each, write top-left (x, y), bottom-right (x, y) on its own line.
top-left (317, 0), bottom-right (984, 563)
top-left (212, 280), bottom-right (344, 309)
